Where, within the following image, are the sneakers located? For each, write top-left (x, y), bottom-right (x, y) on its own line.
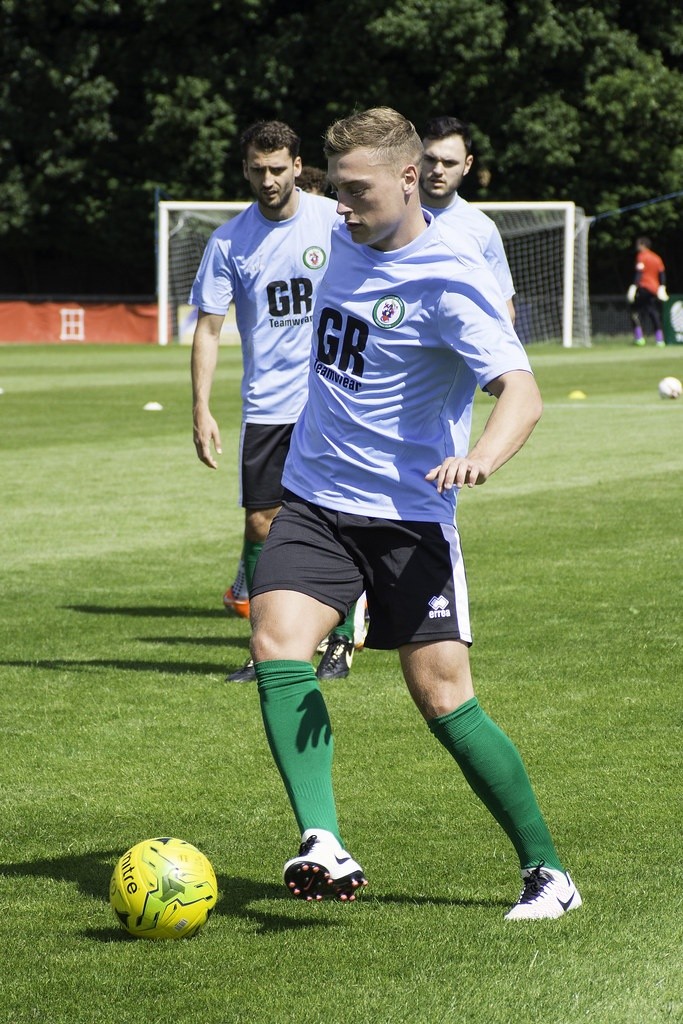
top-left (504, 860), bottom-right (582, 921)
top-left (223, 586), bottom-right (250, 619)
top-left (315, 634), bottom-right (354, 681)
top-left (225, 657), bottom-right (256, 685)
top-left (282, 829), bottom-right (367, 901)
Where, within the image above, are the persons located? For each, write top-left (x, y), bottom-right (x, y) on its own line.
top-left (189, 115), bottom-right (353, 614)
top-left (412, 109), bottom-right (516, 333)
top-left (631, 237), bottom-right (667, 349)
top-left (243, 103), bottom-right (585, 924)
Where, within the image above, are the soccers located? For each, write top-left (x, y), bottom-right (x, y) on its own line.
top-left (109, 837), bottom-right (217, 940)
top-left (657, 376), bottom-right (683, 400)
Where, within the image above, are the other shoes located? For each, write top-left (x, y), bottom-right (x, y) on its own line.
top-left (353, 627), bottom-right (368, 649)
